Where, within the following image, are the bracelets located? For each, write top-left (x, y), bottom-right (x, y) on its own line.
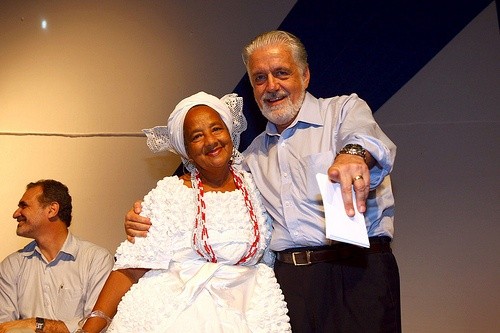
top-left (78, 310), bottom-right (111, 333)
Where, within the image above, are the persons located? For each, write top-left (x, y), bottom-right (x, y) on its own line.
top-left (82, 91), bottom-right (290, 333)
top-left (125, 31), bottom-right (401, 333)
top-left (0, 180), bottom-right (116, 333)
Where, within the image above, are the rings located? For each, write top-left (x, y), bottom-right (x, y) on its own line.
top-left (354, 175), bottom-right (363, 180)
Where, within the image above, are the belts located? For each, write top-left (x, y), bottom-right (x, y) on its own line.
top-left (276, 240), bottom-right (392, 265)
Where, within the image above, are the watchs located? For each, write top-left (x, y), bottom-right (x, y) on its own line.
top-left (335, 144), bottom-right (366, 162)
top-left (35, 317), bottom-right (44, 333)
